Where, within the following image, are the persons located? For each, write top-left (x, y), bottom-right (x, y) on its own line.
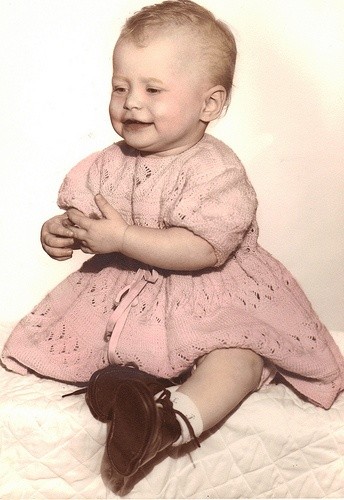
top-left (0, 0), bottom-right (344, 479)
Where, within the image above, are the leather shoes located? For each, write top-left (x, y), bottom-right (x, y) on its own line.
top-left (106, 380), bottom-right (201, 478)
top-left (62, 362), bottom-right (175, 422)
